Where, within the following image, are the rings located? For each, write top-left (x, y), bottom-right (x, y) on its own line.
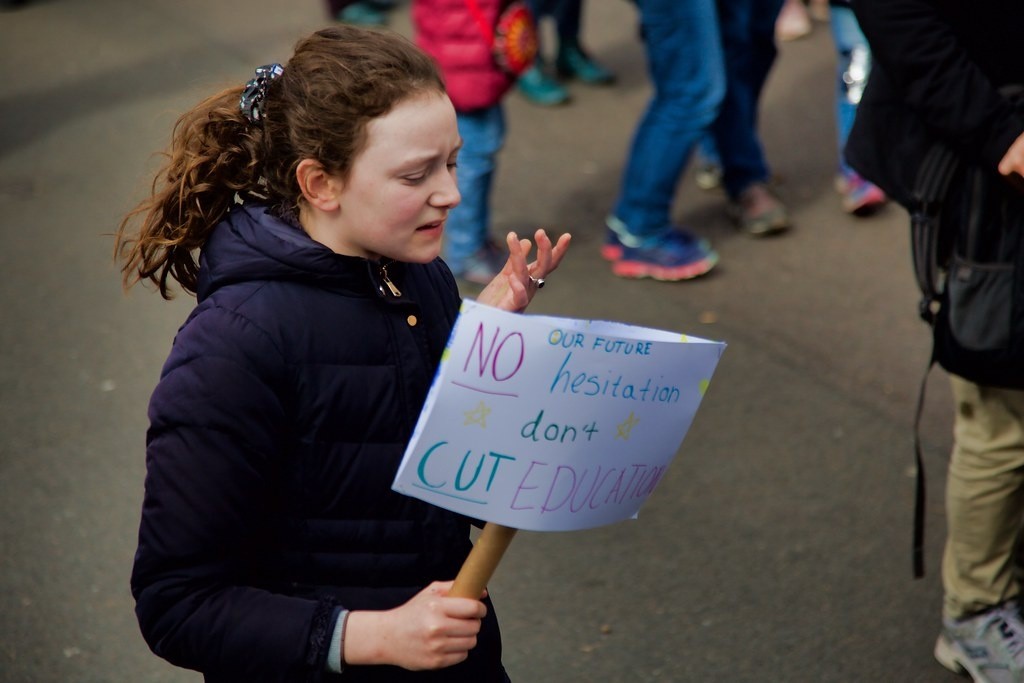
top-left (528, 275), bottom-right (545, 289)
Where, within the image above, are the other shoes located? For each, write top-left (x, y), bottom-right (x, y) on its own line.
top-left (341, 4), bottom-right (387, 25)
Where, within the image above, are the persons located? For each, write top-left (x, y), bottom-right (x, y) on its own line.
top-left (834, 1), bottom-right (888, 213)
top-left (841, 1), bottom-right (1024, 683)
top-left (599, 0), bottom-right (732, 281)
top-left (324, 0), bottom-right (620, 285)
top-left (697, 0), bottom-right (797, 235)
top-left (99, 26), bottom-right (574, 682)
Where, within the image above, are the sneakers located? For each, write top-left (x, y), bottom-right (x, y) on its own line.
top-left (728, 183), bottom-right (793, 232)
top-left (460, 244), bottom-right (505, 286)
top-left (556, 50), bottom-right (615, 89)
top-left (519, 67), bottom-right (570, 105)
top-left (832, 169), bottom-right (888, 215)
top-left (601, 221), bottom-right (718, 281)
top-left (933, 599), bottom-right (1024, 683)
